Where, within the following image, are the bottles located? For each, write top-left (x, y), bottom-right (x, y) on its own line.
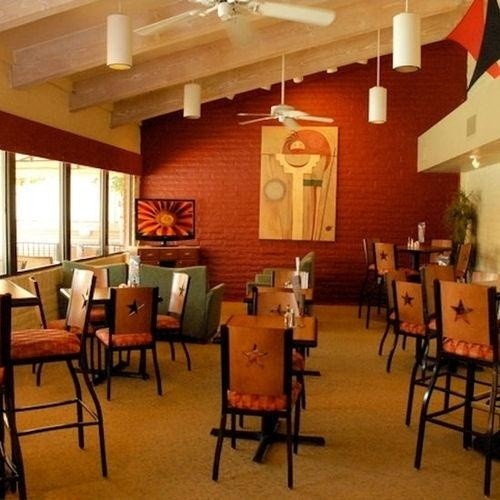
top-left (408, 237), bottom-right (414, 247)
top-left (283, 306), bottom-right (296, 328)
top-left (466, 268), bottom-right (473, 283)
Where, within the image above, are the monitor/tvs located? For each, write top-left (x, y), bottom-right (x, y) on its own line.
top-left (133, 197), bottom-right (197, 248)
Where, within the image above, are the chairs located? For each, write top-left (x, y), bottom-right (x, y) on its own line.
top-left (54, 239), bottom-right (500, 322)
top-left (378, 272), bottom-right (409, 356)
top-left (158, 270), bottom-right (192, 371)
top-left (415, 278), bottom-right (498, 497)
top-left (211, 325), bottom-right (303, 490)
top-left (95, 285), bottom-right (163, 402)
top-left (385, 278), bottom-right (436, 380)
top-left (2, 269), bottom-right (107, 498)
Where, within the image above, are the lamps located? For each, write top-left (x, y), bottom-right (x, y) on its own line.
top-left (368, 30), bottom-right (387, 124)
top-left (184, 80), bottom-right (202, 119)
top-left (393, 0), bottom-right (422, 73)
top-left (106, 0), bottom-right (133, 70)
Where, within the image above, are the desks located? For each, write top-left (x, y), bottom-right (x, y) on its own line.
top-left (1, 278), bottom-right (41, 308)
top-left (209, 313), bottom-right (327, 448)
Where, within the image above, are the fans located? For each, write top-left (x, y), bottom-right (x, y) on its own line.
top-left (236, 55), bottom-right (335, 130)
top-left (133, 0), bottom-right (337, 40)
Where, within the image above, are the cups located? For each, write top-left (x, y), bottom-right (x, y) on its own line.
top-left (457, 278), bottom-right (464, 283)
